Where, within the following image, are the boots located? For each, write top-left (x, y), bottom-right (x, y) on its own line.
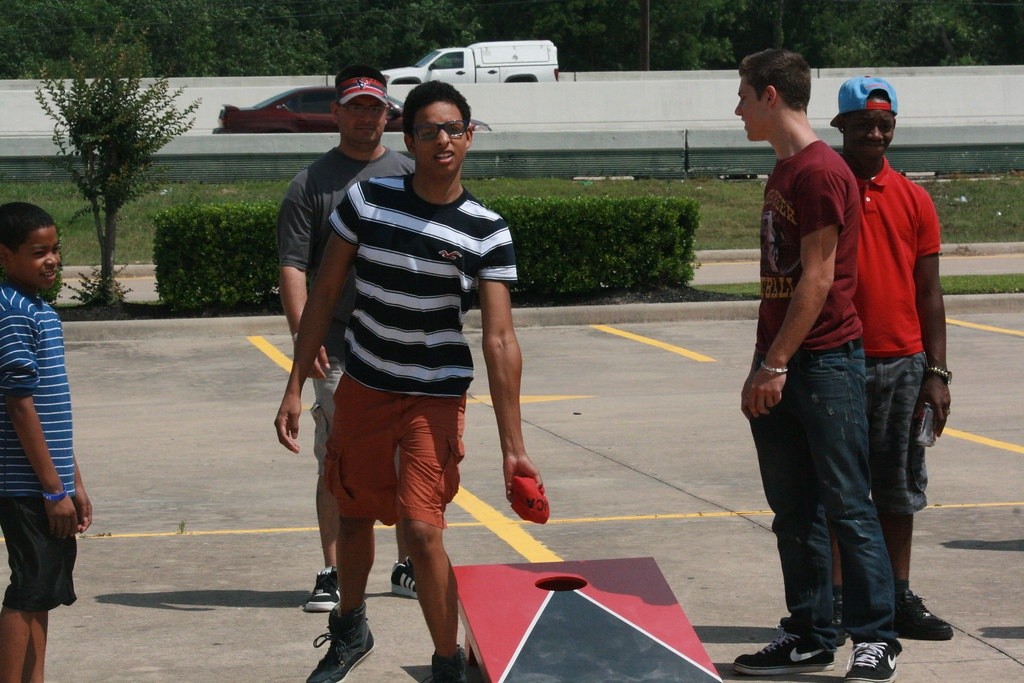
top-left (422, 644), bottom-right (469, 683)
top-left (306, 602), bottom-right (376, 683)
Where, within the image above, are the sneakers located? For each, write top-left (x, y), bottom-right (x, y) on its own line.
top-left (390, 556), bottom-right (418, 599)
top-left (732, 626), bottom-right (835, 674)
top-left (843, 639), bottom-right (903, 683)
top-left (303, 565), bottom-right (340, 612)
top-left (893, 589), bottom-right (954, 641)
top-left (828, 587), bottom-right (846, 646)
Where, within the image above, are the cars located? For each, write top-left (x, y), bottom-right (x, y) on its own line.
top-left (212, 85), bottom-right (493, 132)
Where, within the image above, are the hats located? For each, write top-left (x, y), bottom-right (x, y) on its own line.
top-left (830, 75), bottom-right (898, 127)
top-left (335, 77), bottom-right (388, 106)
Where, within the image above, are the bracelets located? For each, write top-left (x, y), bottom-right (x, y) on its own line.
top-left (762, 361), bottom-right (788, 375)
top-left (292, 334), bottom-right (297, 342)
top-left (43, 482), bottom-right (67, 501)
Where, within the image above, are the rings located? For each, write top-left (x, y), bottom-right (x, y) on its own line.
top-left (944, 408), bottom-right (950, 416)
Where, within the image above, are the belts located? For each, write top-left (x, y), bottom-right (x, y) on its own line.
top-left (810, 337), bottom-right (864, 356)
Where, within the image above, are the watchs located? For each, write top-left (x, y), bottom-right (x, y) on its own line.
top-left (926, 366), bottom-right (952, 386)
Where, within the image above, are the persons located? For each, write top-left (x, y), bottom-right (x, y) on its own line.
top-left (823, 74), bottom-right (952, 645)
top-left (0, 202), bottom-right (92, 683)
top-left (274, 80), bottom-right (543, 683)
top-left (735, 49), bottom-right (903, 683)
top-left (277, 65), bottom-right (415, 613)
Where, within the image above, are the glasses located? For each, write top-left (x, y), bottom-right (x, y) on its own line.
top-left (339, 103), bottom-right (388, 118)
top-left (413, 119), bottom-right (469, 141)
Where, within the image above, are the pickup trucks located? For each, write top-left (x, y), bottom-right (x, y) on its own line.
top-left (380, 39), bottom-right (560, 84)
